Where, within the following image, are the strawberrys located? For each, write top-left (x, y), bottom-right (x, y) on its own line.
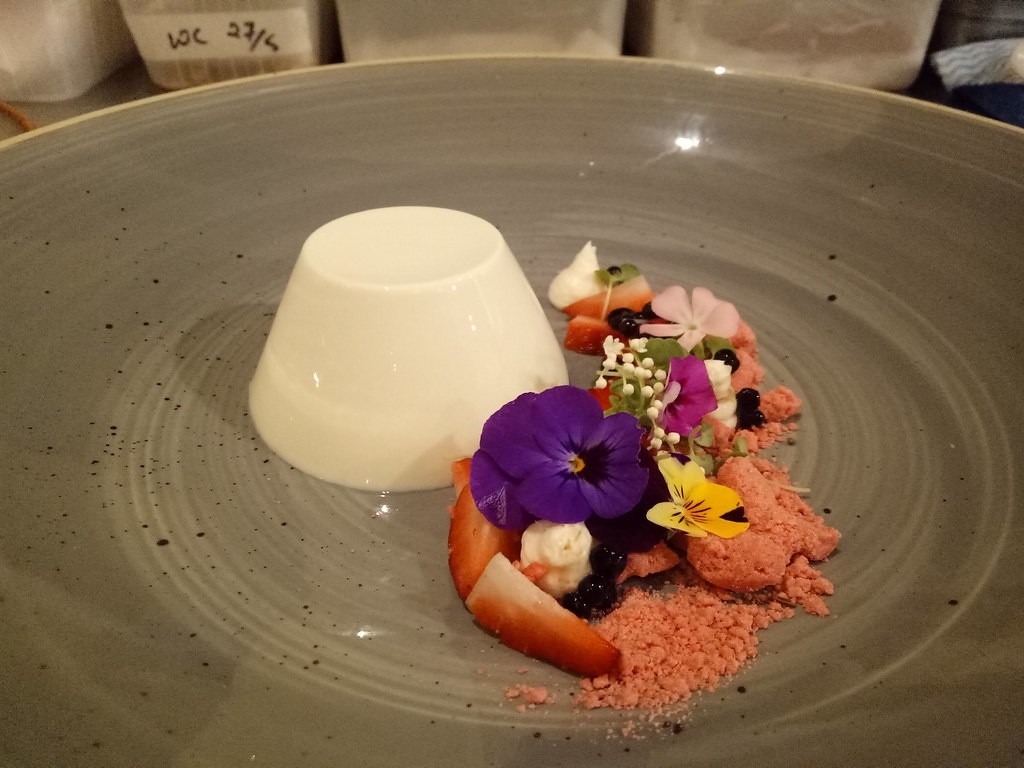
top-left (448, 458), bottom-right (621, 678)
top-left (561, 276), bottom-right (671, 411)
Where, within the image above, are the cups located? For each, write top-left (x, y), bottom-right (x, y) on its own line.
top-left (627, 1), bottom-right (941, 92)
top-left (68, 1), bottom-right (137, 97)
top-left (336, 2), bottom-right (626, 63)
top-left (115, 2), bottom-right (335, 85)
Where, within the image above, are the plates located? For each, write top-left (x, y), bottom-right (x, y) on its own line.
top-left (1, 55), bottom-right (1022, 767)
